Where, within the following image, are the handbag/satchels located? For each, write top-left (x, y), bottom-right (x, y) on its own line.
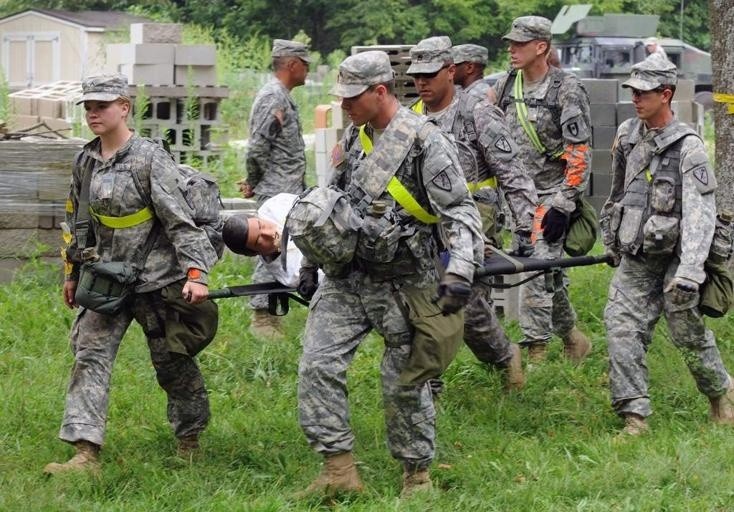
top-left (700, 260), bottom-right (734, 318)
top-left (565, 198), bottom-right (599, 258)
top-left (76, 261), bottom-right (135, 315)
top-left (161, 278), bottom-right (217, 356)
top-left (282, 184), bottom-right (434, 276)
top-left (400, 285), bottom-right (464, 385)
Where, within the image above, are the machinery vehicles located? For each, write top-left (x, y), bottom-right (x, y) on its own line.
top-left (482, 6), bottom-right (714, 129)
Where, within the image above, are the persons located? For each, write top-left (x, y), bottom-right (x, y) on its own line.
top-left (235, 38), bottom-right (312, 342)
top-left (43, 73), bottom-right (218, 477)
top-left (647, 37), bottom-right (666, 60)
top-left (489, 15), bottom-right (592, 370)
top-left (223, 192), bottom-right (326, 290)
top-left (600, 50), bottom-right (734, 437)
top-left (286, 50), bottom-right (480, 503)
top-left (381, 35), bottom-right (523, 396)
top-left (450, 43), bottom-right (491, 103)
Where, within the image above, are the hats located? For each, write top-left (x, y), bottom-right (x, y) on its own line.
top-left (272, 39), bottom-right (312, 63)
top-left (328, 51), bottom-right (393, 98)
top-left (622, 58), bottom-right (677, 91)
top-left (406, 36), bottom-right (488, 73)
top-left (75, 75), bottom-right (129, 104)
top-left (502, 16), bottom-right (552, 42)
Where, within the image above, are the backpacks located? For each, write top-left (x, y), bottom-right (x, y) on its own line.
top-left (134, 137), bottom-right (224, 259)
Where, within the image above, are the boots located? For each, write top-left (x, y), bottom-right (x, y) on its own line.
top-left (290, 452), bottom-right (362, 498)
top-left (712, 377), bottom-right (734, 424)
top-left (44, 442), bottom-right (101, 476)
top-left (504, 330), bottom-right (591, 388)
top-left (250, 309), bottom-right (286, 341)
top-left (401, 466), bottom-right (432, 499)
top-left (615, 415), bottom-right (645, 442)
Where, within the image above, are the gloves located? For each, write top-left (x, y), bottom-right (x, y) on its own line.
top-left (606, 247), bottom-right (620, 267)
top-left (542, 207), bottom-right (568, 243)
top-left (663, 277), bottom-right (699, 305)
top-left (431, 272), bottom-right (471, 315)
top-left (298, 269), bottom-right (319, 300)
